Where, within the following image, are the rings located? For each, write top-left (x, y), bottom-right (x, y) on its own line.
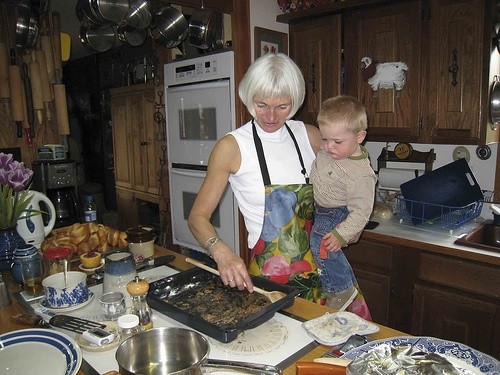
top-left (228, 279), bottom-right (234, 282)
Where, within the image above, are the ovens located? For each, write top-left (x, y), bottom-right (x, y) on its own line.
top-left (164, 51), bottom-right (241, 258)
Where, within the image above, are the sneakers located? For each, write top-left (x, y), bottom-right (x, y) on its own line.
top-left (324, 285), bottom-right (357, 312)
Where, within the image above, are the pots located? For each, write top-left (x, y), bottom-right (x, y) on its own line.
top-left (75, 0), bottom-right (153, 53)
top-left (115, 327), bottom-right (282, 375)
top-left (188, 0), bottom-right (218, 52)
top-left (11, 0), bottom-right (40, 49)
top-left (148, 0), bottom-right (188, 49)
top-left (489, 75), bottom-right (500, 131)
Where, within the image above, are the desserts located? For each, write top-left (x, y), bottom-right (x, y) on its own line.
top-left (80, 250), bottom-right (102, 268)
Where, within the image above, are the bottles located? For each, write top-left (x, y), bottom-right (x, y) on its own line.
top-left (41, 247), bottom-right (71, 280)
top-left (117, 315), bottom-right (140, 344)
top-left (126, 279), bottom-right (153, 335)
top-left (83, 196), bottom-right (96, 223)
top-left (53, 189), bottom-right (76, 220)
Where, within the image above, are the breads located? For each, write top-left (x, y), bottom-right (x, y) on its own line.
top-left (372, 205), bottom-right (393, 220)
top-left (39, 222), bottom-right (129, 257)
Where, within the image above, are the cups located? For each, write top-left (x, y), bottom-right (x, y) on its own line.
top-left (23, 259), bottom-right (42, 297)
top-left (80, 252), bottom-right (101, 268)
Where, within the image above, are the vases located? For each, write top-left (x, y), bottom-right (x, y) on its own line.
top-left (0, 223), bottom-right (26, 271)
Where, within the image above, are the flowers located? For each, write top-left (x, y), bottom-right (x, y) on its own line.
top-left (0, 152), bottom-right (51, 229)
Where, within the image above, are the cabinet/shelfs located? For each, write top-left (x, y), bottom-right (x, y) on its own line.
top-left (276, 0), bottom-right (490, 145)
top-left (110, 82), bottom-right (167, 204)
top-left (342, 237), bottom-right (500, 361)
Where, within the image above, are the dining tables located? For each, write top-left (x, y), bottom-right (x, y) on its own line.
top-left (0, 242), bottom-right (412, 375)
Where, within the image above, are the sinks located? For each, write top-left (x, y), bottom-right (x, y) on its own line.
top-left (454, 219), bottom-right (500, 253)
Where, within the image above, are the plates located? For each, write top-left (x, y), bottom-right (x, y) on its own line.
top-left (0, 328), bottom-right (83, 375)
top-left (39, 289), bottom-right (94, 312)
top-left (339, 337), bottom-right (500, 375)
top-left (79, 259), bottom-right (105, 272)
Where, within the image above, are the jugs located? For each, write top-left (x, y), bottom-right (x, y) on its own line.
top-left (10, 244), bottom-right (40, 289)
top-left (103, 248), bottom-right (138, 315)
top-left (124, 225), bottom-right (157, 260)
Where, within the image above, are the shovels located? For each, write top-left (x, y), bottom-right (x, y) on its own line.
top-left (10, 311), bottom-right (107, 336)
top-left (184, 257), bottom-right (287, 304)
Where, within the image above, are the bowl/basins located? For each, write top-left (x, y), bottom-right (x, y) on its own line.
top-left (42, 271), bottom-right (87, 309)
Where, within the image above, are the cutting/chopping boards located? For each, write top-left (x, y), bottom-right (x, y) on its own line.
top-left (400, 158), bottom-right (484, 225)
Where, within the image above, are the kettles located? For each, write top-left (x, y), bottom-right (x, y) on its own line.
top-left (11, 190), bottom-right (56, 249)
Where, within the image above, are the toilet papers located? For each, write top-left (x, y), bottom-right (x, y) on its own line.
top-left (378, 168), bottom-right (425, 190)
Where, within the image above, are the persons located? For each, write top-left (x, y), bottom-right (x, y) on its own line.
top-left (187, 52), bottom-right (373, 324)
top-left (306, 96), bottom-right (378, 313)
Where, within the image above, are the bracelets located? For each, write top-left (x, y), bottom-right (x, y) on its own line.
top-left (205, 237), bottom-right (223, 253)
